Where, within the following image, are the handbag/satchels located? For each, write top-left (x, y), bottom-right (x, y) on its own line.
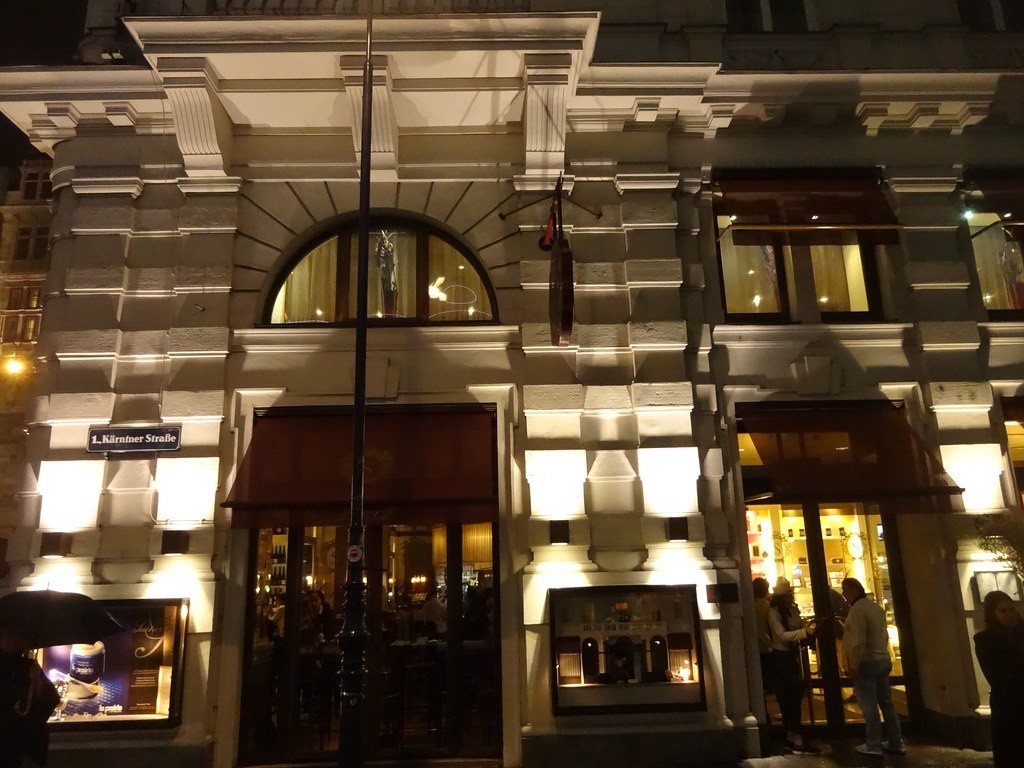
top-left (24, 723), bottom-right (49, 766)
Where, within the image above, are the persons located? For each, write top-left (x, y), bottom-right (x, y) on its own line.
top-left (973, 591), bottom-right (1024, 768)
top-left (828, 584), bottom-right (856, 703)
top-left (300, 590), bottom-right (341, 721)
top-left (753, 576), bottom-right (821, 755)
top-left (424, 587), bottom-right (495, 639)
top-left (841, 578), bottom-right (906, 757)
top-left (0, 655), bottom-right (60, 768)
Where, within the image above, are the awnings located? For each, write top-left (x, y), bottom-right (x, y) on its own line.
top-left (220, 408), bottom-right (500, 530)
top-left (965, 169), bottom-right (1024, 243)
top-left (712, 166), bottom-right (901, 245)
top-left (737, 398), bottom-right (966, 515)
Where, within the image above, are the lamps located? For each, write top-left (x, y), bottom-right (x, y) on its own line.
top-left (665, 516), bottom-right (689, 543)
top-left (39, 532), bottom-right (72, 561)
top-left (549, 520), bottom-right (570, 546)
top-left (162, 530), bottom-right (190, 558)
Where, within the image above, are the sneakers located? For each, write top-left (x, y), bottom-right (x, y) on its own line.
top-left (792, 743), bottom-right (821, 756)
top-left (783, 739), bottom-right (810, 750)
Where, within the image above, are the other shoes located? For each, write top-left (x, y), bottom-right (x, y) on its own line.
top-left (881, 739), bottom-right (906, 753)
top-left (856, 743), bottom-right (883, 755)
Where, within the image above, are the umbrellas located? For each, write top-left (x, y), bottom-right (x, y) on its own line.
top-left (0, 590), bottom-right (126, 714)
